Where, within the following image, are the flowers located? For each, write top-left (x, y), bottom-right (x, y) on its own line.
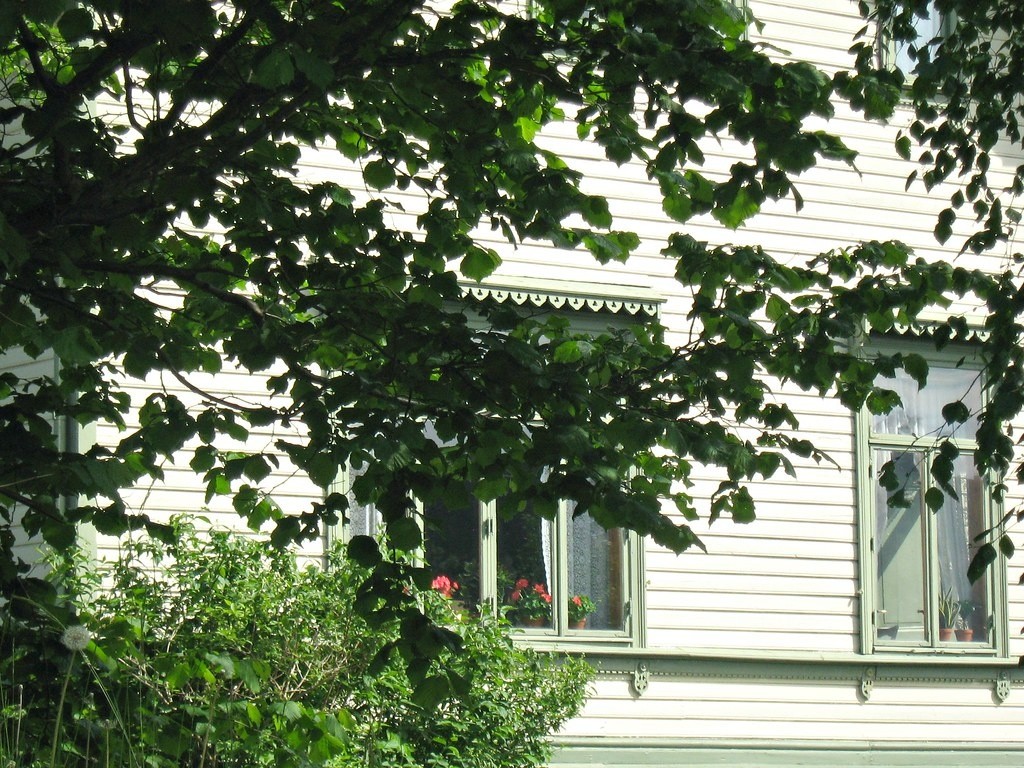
top-left (512, 579), bottom-right (552, 622)
top-left (568, 593), bottom-right (596, 624)
top-left (432, 576), bottom-right (458, 599)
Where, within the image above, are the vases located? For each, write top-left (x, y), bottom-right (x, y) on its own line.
top-left (569, 618), bottom-right (586, 629)
top-left (522, 614), bottom-right (545, 627)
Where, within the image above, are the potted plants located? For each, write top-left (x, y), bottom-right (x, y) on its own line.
top-left (954, 600), bottom-right (982, 642)
top-left (940, 585), bottom-right (960, 641)
